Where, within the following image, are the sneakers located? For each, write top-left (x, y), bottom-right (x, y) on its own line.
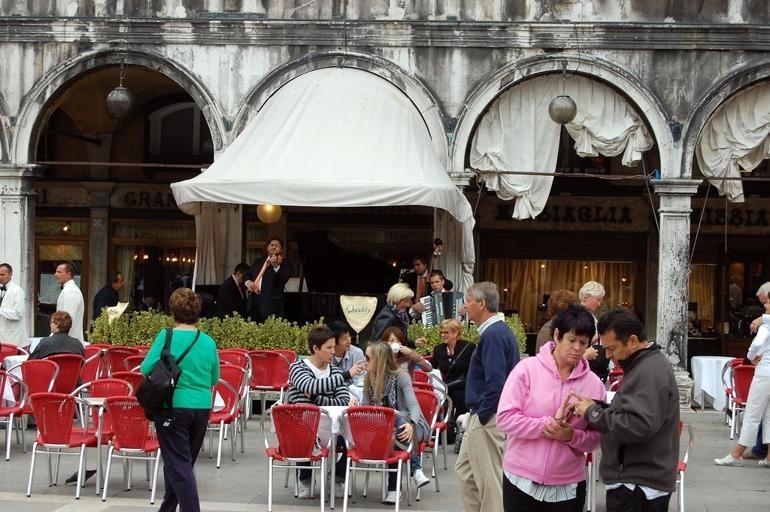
top-left (414, 468), bottom-right (430, 489)
top-left (298, 485), bottom-right (312, 499)
top-left (328, 470), bottom-right (346, 499)
top-left (714, 453), bottom-right (770, 468)
top-left (385, 492), bottom-right (403, 504)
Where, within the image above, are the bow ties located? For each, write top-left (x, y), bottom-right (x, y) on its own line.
top-left (59, 284), bottom-right (63, 290)
top-left (0, 287), bottom-right (7, 291)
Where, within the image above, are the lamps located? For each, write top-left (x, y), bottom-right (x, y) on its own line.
top-left (104, 62), bottom-right (136, 119)
top-left (256, 204), bottom-right (282, 224)
top-left (548, 70), bottom-right (578, 124)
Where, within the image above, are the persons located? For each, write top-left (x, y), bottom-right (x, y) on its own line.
top-left (49, 263), bottom-right (85, 340)
top-left (0, 263), bottom-right (31, 351)
top-left (89, 270), bottom-right (126, 333)
top-left (27, 311), bottom-right (85, 363)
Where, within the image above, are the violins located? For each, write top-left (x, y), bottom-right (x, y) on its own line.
top-left (267, 253), bottom-right (282, 266)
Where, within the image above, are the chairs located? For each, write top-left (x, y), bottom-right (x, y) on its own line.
top-left (589, 355), bottom-right (761, 512)
top-left (337, 407), bottom-right (413, 511)
top-left (1, 341), bottom-right (254, 489)
top-left (262, 403), bottom-right (335, 511)
top-left (101, 395), bottom-right (165, 505)
top-left (26, 392), bottom-right (104, 500)
top-left (248, 349), bottom-right (452, 501)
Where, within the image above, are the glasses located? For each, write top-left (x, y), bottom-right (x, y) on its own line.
top-left (440, 330), bottom-right (451, 335)
top-left (47, 320), bottom-right (54, 324)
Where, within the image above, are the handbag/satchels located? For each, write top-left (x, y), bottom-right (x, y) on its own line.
top-left (587, 344), bottom-right (610, 386)
top-left (136, 329), bottom-right (181, 419)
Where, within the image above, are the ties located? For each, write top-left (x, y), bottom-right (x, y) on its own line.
top-left (418, 277), bottom-right (425, 302)
